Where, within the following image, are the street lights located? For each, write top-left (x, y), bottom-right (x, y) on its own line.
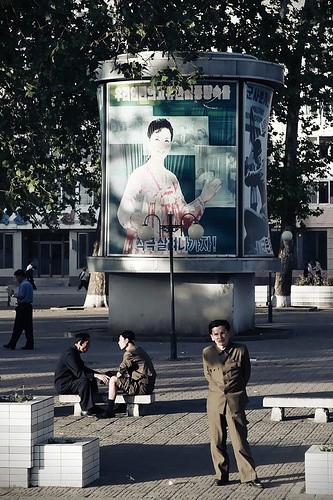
top-left (137, 213), bottom-right (205, 363)
top-left (267, 225), bottom-right (293, 322)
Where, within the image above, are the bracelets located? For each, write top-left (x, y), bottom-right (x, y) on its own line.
top-left (12, 294), bottom-right (14, 297)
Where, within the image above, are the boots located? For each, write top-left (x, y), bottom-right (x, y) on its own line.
top-left (114, 404), bottom-right (127, 412)
top-left (96, 400), bottom-right (115, 418)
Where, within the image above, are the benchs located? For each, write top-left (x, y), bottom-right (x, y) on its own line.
top-left (262, 396), bottom-right (333, 423)
top-left (58, 392), bottom-right (155, 417)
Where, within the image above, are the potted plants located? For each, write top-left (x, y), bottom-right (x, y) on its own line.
top-left (0, 385), bottom-right (55, 488)
top-left (305, 443), bottom-right (333, 495)
top-left (32, 437), bottom-right (100, 489)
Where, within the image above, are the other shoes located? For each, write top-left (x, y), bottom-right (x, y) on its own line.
top-left (3, 345), bottom-right (15, 350)
top-left (81, 411), bottom-right (94, 418)
top-left (21, 346), bottom-right (34, 350)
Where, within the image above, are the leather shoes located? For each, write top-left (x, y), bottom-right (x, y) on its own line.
top-left (246, 479), bottom-right (263, 488)
top-left (215, 480), bottom-right (228, 486)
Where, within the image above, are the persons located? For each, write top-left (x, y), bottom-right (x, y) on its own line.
top-left (115, 118), bottom-right (264, 256)
top-left (76, 267), bottom-right (90, 291)
top-left (54, 333), bottom-right (110, 418)
top-left (3, 269), bottom-right (35, 350)
top-left (95, 331), bottom-right (156, 419)
top-left (25, 262), bottom-right (37, 290)
top-left (308, 261), bottom-right (326, 284)
top-left (202, 319), bottom-right (264, 489)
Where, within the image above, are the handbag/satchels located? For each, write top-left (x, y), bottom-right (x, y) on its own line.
top-left (9, 295), bottom-right (18, 306)
